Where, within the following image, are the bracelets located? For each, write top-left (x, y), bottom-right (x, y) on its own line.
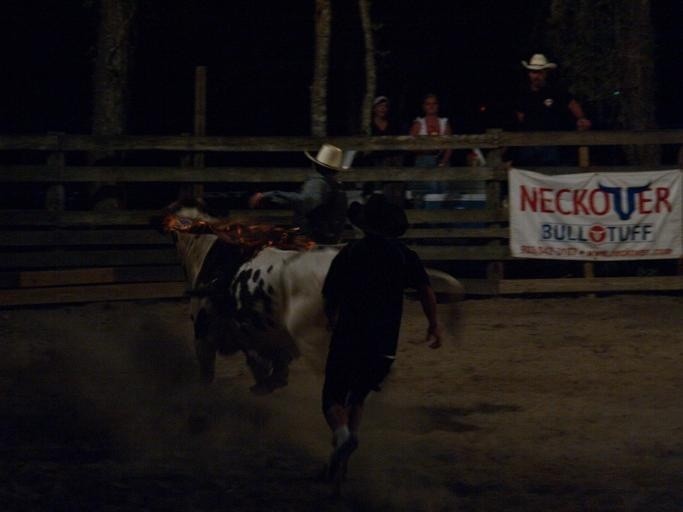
top-left (438, 158), bottom-right (449, 165)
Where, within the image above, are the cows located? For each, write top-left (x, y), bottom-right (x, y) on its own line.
top-left (160, 196), bottom-right (465, 397)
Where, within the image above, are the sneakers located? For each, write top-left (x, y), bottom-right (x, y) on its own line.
top-left (248, 370), bottom-right (287, 396)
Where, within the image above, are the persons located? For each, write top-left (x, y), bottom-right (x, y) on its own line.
top-left (410, 93), bottom-right (453, 167)
top-left (514, 52), bottom-right (593, 132)
top-left (367, 92), bottom-right (410, 169)
top-left (247, 142), bottom-right (354, 397)
top-left (319, 193), bottom-right (443, 483)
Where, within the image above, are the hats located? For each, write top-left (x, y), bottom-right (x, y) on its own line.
top-left (348, 193), bottom-right (409, 237)
top-left (521, 54), bottom-right (558, 70)
top-left (304, 143), bottom-right (350, 173)
